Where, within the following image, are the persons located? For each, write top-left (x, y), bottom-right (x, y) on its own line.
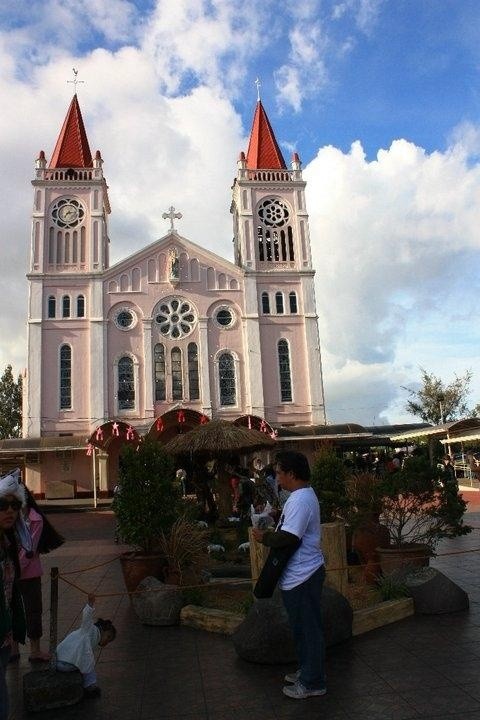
top-left (232, 465), bottom-right (256, 541)
top-left (393, 454), bottom-right (400, 467)
top-left (194, 471), bottom-right (217, 514)
top-left (56, 592), bottom-right (117, 696)
top-left (443, 455), bottom-right (455, 480)
top-left (6, 483), bottom-right (52, 663)
top-left (252, 451), bottom-right (328, 699)
top-left (468, 453), bottom-right (480, 482)
top-left (0, 466), bottom-right (26, 720)
top-left (176, 466), bottom-right (187, 497)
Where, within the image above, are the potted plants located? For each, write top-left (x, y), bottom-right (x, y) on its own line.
top-left (109, 433), bottom-right (190, 602)
top-left (309, 436), bottom-right (476, 579)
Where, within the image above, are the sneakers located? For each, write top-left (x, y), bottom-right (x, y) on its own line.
top-left (29, 653), bottom-right (51, 663)
top-left (283, 670), bottom-right (326, 698)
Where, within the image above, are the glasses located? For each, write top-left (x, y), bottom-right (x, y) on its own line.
top-left (0, 499), bottom-right (23, 511)
